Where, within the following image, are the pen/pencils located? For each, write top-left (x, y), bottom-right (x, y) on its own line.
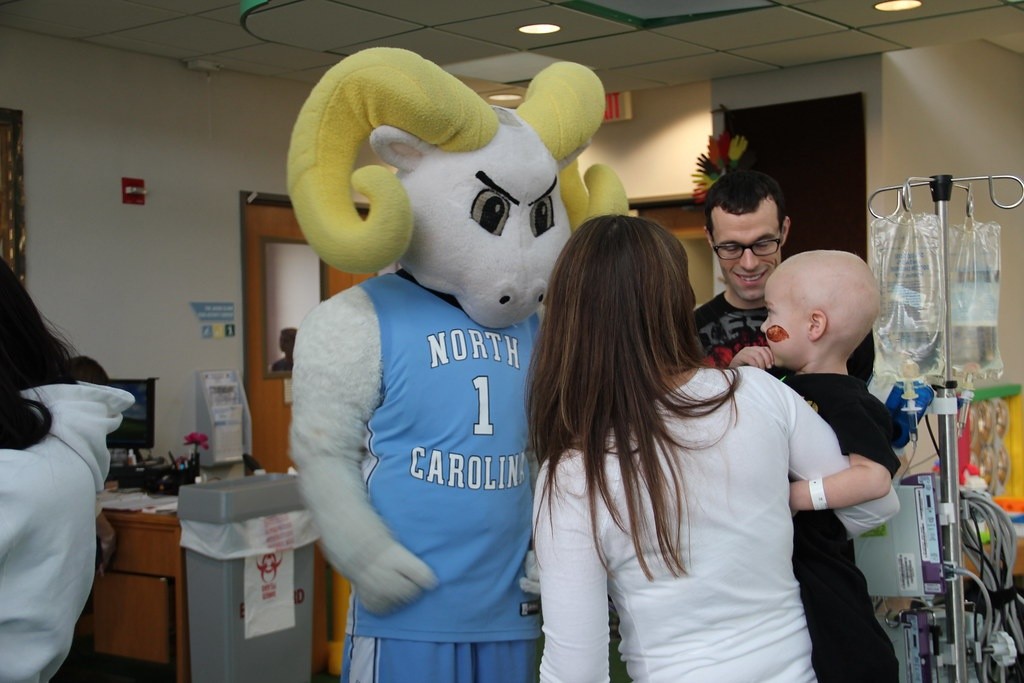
top-left (167, 451), bottom-right (194, 474)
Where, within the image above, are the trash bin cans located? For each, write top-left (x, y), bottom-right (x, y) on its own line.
top-left (176, 470), bottom-right (320, 683)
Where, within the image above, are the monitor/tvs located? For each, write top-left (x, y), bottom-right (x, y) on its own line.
top-left (106, 377), bottom-right (156, 464)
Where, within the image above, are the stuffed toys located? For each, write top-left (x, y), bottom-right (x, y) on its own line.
top-left (289, 46), bottom-right (628, 683)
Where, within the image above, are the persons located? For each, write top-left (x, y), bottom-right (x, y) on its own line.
top-left (272, 328), bottom-right (296, 371)
top-left (528, 215), bottom-right (900, 683)
top-left (1, 256), bottom-right (134, 682)
top-left (727, 251), bottom-right (901, 683)
top-left (690, 172), bottom-right (874, 385)
top-left (65, 356), bottom-right (115, 577)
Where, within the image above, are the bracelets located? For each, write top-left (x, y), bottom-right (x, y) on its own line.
top-left (808, 477), bottom-right (828, 511)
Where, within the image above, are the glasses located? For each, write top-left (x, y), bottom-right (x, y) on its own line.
top-left (712, 228), bottom-right (781, 260)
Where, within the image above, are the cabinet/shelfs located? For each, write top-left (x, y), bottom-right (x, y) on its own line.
top-left (75, 507), bottom-right (189, 683)
